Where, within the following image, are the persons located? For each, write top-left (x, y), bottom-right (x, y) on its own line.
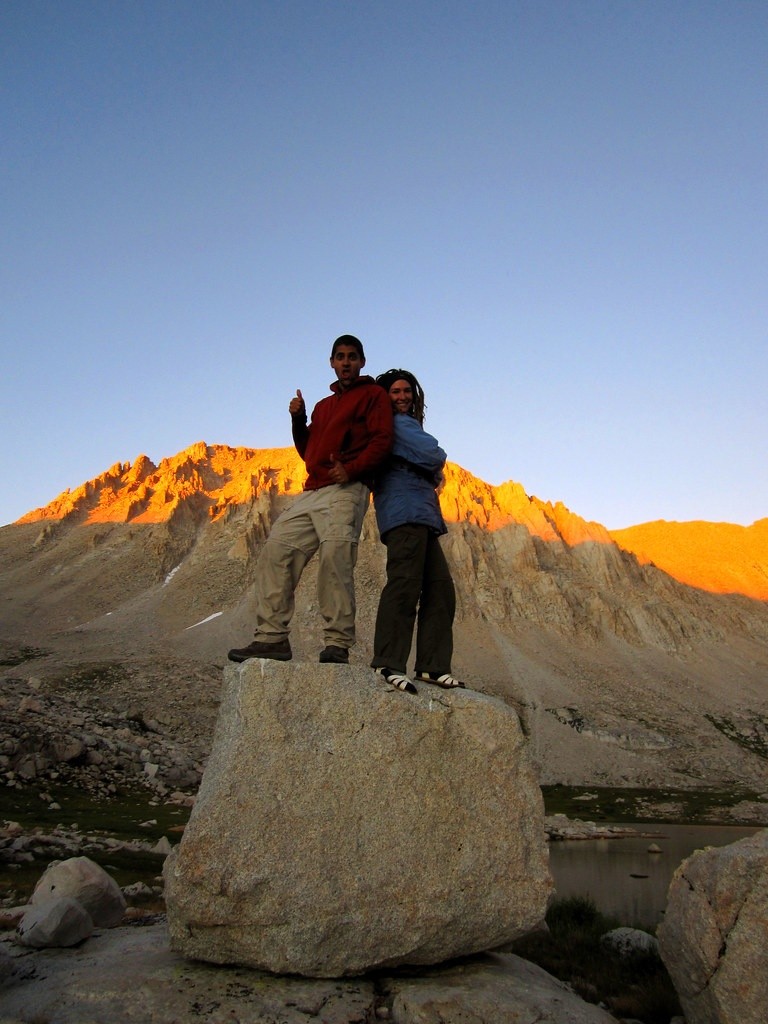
top-left (227, 334), bottom-right (395, 663)
top-left (372, 368), bottom-right (466, 696)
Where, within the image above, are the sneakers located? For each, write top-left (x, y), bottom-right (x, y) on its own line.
top-left (319, 644), bottom-right (349, 664)
top-left (228, 637), bottom-right (293, 663)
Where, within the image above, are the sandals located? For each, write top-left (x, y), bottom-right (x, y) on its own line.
top-left (375, 668), bottom-right (418, 695)
top-left (413, 671), bottom-right (466, 688)
